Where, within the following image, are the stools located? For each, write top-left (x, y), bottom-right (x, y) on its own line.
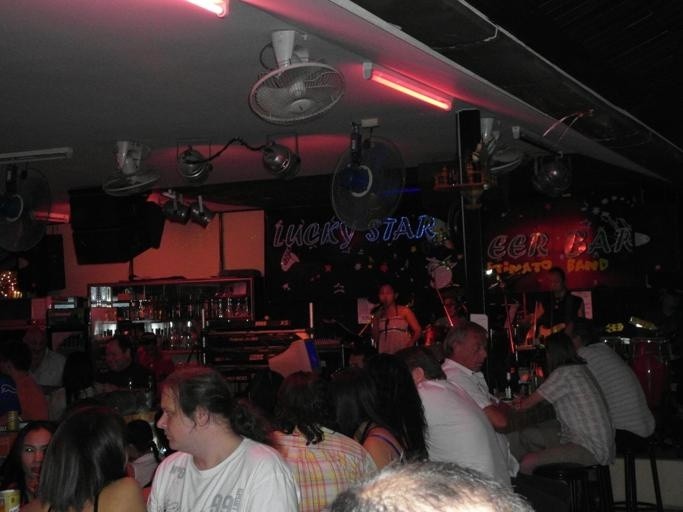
top-left (533, 462), bottom-right (587, 512)
top-left (579, 464), bottom-right (614, 512)
top-left (614, 431), bottom-right (665, 508)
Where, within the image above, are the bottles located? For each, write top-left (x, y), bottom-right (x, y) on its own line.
top-left (130, 295), bottom-right (169, 323)
top-left (236, 299), bottom-right (242, 320)
top-left (210, 299), bottom-right (214, 320)
top-left (226, 299), bottom-right (234, 320)
top-left (218, 298), bottom-right (223, 320)
top-left (175, 297), bottom-right (181, 321)
top-left (244, 298), bottom-right (250, 320)
top-left (187, 294), bottom-right (193, 322)
top-left (504, 369), bottom-right (513, 401)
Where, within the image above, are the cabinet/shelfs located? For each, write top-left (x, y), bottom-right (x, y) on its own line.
top-left (201, 303), bottom-right (314, 398)
top-left (87, 273), bottom-right (264, 357)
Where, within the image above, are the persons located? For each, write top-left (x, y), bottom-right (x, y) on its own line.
top-left (244, 367), bottom-right (290, 437)
top-left (262, 371), bottom-right (380, 512)
top-left (391, 348), bottom-right (511, 491)
top-left (441, 323), bottom-right (572, 512)
top-left (21, 326), bottom-right (80, 398)
top-left (104, 336), bottom-right (157, 411)
top-left (122, 419), bottom-right (161, 487)
top-left (133, 333), bottom-right (176, 380)
top-left (372, 282), bottom-right (420, 353)
top-left (49, 352), bottom-right (147, 422)
top-left (326, 371), bottom-right (378, 444)
top-left (508, 332), bottom-right (615, 478)
top-left (0, 420), bottom-right (56, 505)
top-left (328, 461), bottom-right (534, 512)
top-left (436, 296), bottom-right (467, 333)
top-left (349, 346), bottom-right (375, 371)
top-left (21, 406), bottom-right (146, 512)
top-left (526, 268), bottom-right (584, 339)
top-left (147, 366), bottom-right (302, 512)
top-left (564, 318), bottom-right (654, 455)
top-left (7, 340), bottom-right (49, 423)
top-left (0, 349), bottom-right (21, 427)
top-left (367, 353), bottom-right (428, 463)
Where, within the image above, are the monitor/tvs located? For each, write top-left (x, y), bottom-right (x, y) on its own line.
top-left (268, 339), bottom-right (322, 377)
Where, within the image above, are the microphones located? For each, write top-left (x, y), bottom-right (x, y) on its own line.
top-left (369, 301), bottom-right (387, 314)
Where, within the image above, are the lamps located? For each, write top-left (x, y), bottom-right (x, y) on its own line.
top-left (262, 144), bottom-right (302, 178)
top-left (0, 147), bottom-right (74, 161)
top-left (363, 59), bottom-right (453, 113)
top-left (161, 188), bottom-right (192, 226)
top-left (174, 144), bottom-right (212, 186)
top-left (188, 194), bottom-right (214, 227)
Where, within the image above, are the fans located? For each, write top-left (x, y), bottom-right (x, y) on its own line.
top-left (0, 163), bottom-right (53, 253)
top-left (327, 116), bottom-right (409, 235)
top-left (102, 141), bottom-right (163, 197)
top-left (472, 118), bottom-right (522, 175)
top-left (248, 31), bottom-right (346, 126)
